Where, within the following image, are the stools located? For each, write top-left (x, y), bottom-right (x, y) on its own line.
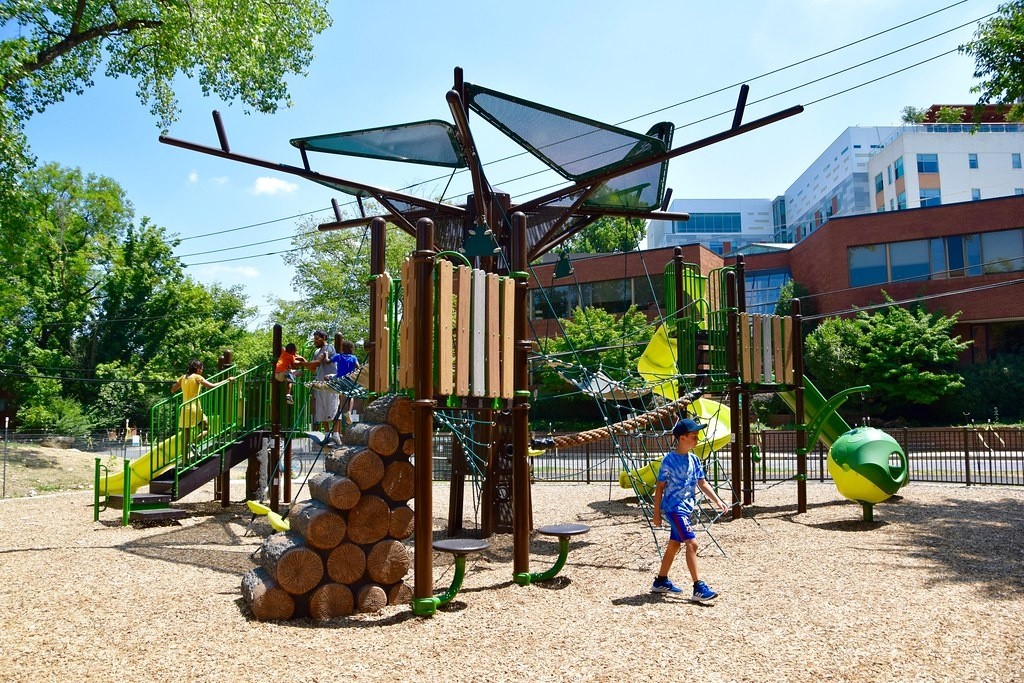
top-left (410, 538), bottom-right (491, 615)
top-left (513, 524), bottom-right (590, 586)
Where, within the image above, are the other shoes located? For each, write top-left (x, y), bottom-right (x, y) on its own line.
top-left (287, 395), bottom-right (294, 404)
top-left (185, 463), bottom-right (199, 470)
top-left (191, 446), bottom-right (200, 457)
top-left (332, 435), bottom-right (342, 446)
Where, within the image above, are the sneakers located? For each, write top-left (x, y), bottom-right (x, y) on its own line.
top-left (692, 581), bottom-right (718, 600)
top-left (651, 577), bottom-right (683, 594)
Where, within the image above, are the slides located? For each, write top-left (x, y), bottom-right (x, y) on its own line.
top-left (617, 320), bottom-right (734, 499)
top-left (764, 369), bottom-right (911, 496)
top-left (94, 415), bottom-right (224, 495)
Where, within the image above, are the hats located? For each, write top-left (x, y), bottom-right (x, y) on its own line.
top-left (673, 419), bottom-right (708, 439)
